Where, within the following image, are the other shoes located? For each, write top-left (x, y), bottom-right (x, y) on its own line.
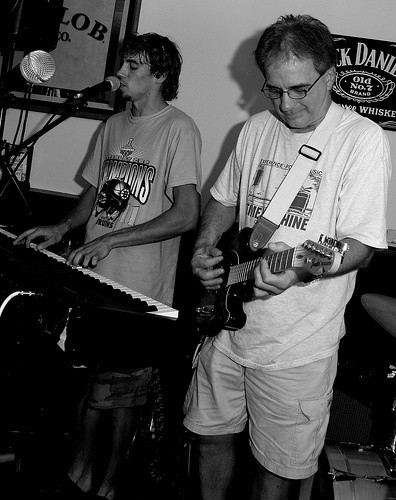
top-left (32, 473), bottom-right (84, 500)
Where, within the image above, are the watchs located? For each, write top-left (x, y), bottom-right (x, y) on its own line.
top-left (295, 275), bottom-right (324, 287)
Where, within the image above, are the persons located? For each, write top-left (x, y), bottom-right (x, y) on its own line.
top-left (12, 32), bottom-right (202, 500)
top-left (183, 13), bottom-right (391, 500)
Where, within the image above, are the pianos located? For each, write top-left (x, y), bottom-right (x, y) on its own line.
top-left (0, 227), bottom-right (179, 500)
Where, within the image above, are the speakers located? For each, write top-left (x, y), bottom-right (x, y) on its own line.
top-left (0, 0), bottom-right (64, 53)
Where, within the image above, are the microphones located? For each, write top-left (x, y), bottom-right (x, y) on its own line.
top-left (73, 76), bottom-right (120, 98)
top-left (0, 49), bottom-right (55, 94)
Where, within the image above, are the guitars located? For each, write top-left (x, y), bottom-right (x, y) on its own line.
top-left (194, 234), bottom-right (350, 336)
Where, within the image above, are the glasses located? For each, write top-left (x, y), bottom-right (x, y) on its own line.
top-left (261, 72), bottom-right (324, 99)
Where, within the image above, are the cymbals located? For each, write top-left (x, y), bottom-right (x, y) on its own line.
top-left (362, 294), bottom-right (396, 337)
